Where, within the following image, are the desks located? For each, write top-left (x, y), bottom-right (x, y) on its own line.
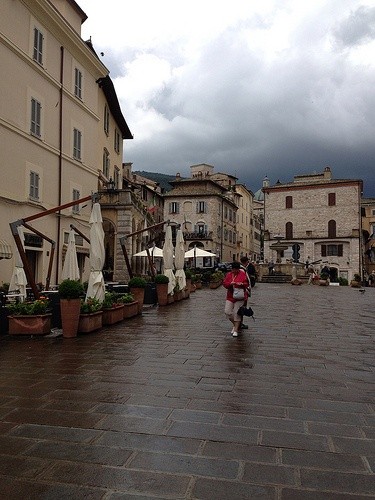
top-left (3, 285), bottom-right (59, 302)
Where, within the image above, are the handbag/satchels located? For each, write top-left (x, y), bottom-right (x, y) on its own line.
top-left (233, 288), bottom-right (244, 298)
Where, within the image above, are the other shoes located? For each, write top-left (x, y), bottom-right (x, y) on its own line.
top-left (233, 332), bottom-right (238, 336)
top-left (231, 327), bottom-right (235, 333)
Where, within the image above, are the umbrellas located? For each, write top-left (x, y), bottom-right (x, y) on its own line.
top-left (8, 226), bottom-right (28, 300)
top-left (183, 247), bottom-right (217, 259)
top-left (85, 203), bottom-right (106, 306)
top-left (174, 224), bottom-right (187, 290)
top-left (133, 247), bottom-right (176, 258)
top-left (163, 224), bottom-right (177, 296)
top-left (62, 230), bottom-right (80, 281)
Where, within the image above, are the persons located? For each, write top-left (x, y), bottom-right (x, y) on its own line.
top-left (268, 260), bottom-right (275, 275)
top-left (224, 262), bottom-right (251, 337)
top-left (240, 257), bottom-right (256, 288)
top-left (309, 270), bottom-right (317, 285)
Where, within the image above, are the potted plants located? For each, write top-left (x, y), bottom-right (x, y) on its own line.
top-left (128, 277), bottom-right (147, 314)
top-left (154, 268), bottom-right (225, 305)
top-left (79, 296), bottom-right (103, 332)
top-left (7, 301), bottom-right (53, 340)
top-left (57, 278), bottom-right (85, 337)
top-left (100, 292), bottom-right (125, 324)
top-left (117, 292), bottom-right (139, 318)
